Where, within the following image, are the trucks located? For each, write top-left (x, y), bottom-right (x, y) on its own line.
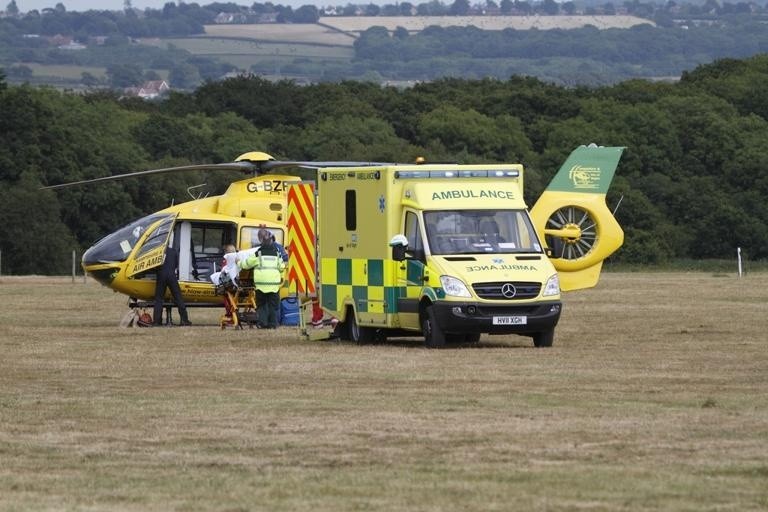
top-left (314, 161), bottom-right (562, 349)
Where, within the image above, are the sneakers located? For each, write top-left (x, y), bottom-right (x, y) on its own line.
top-left (152, 321), bottom-right (161, 326)
top-left (181, 321), bottom-right (191, 324)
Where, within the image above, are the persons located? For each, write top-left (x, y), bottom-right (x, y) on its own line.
top-left (265, 230), bottom-right (290, 327)
top-left (149, 239), bottom-right (193, 326)
top-left (234, 229), bottom-right (286, 329)
top-left (218, 244), bottom-right (240, 326)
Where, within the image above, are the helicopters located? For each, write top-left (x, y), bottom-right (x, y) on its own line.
top-left (38, 140), bottom-right (627, 329)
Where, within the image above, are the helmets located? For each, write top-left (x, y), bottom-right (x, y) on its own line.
top-left (222, 244), bottom-right (236, 253)
top-left (389, 235), bottom-right (408, 249)
